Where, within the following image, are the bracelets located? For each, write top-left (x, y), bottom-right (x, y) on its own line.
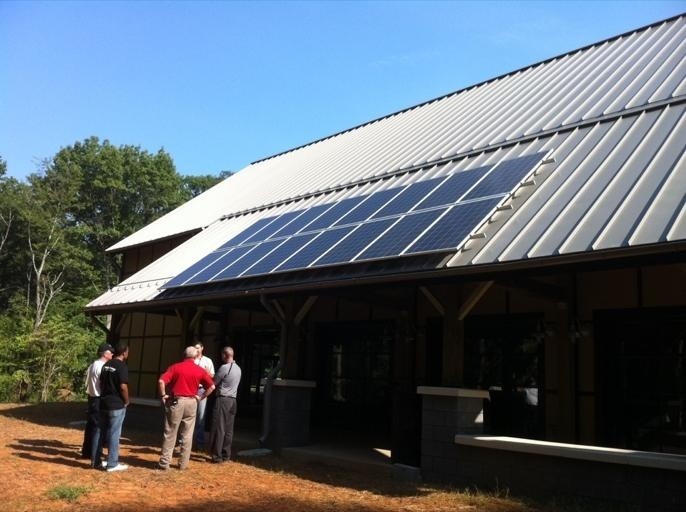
top-left (160, 393), bottom-right (167, 398)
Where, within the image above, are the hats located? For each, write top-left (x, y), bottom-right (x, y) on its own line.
top-left (98, 342), bottom-right (116, 353)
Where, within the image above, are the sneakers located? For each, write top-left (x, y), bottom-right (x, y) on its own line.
top-left (151, 461), bottom-right (168, 470)
top-left (92, 461), bottom-right (108, 469)
top-left (106, 463), bottom-right (129, 472)
top-left (175, 462), bottom-right (186, 469)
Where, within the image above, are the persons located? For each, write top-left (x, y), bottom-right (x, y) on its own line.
top-left (153, 347), bottom-right (216, 471)
top-left (172, 341), bottom-right (214, 456)
top-left (211, 345), bottom-right (242, 464)
top-left (95, 342), bottom-right (130, 472)
top-left (80, 341), bottom-right (114, 458)
